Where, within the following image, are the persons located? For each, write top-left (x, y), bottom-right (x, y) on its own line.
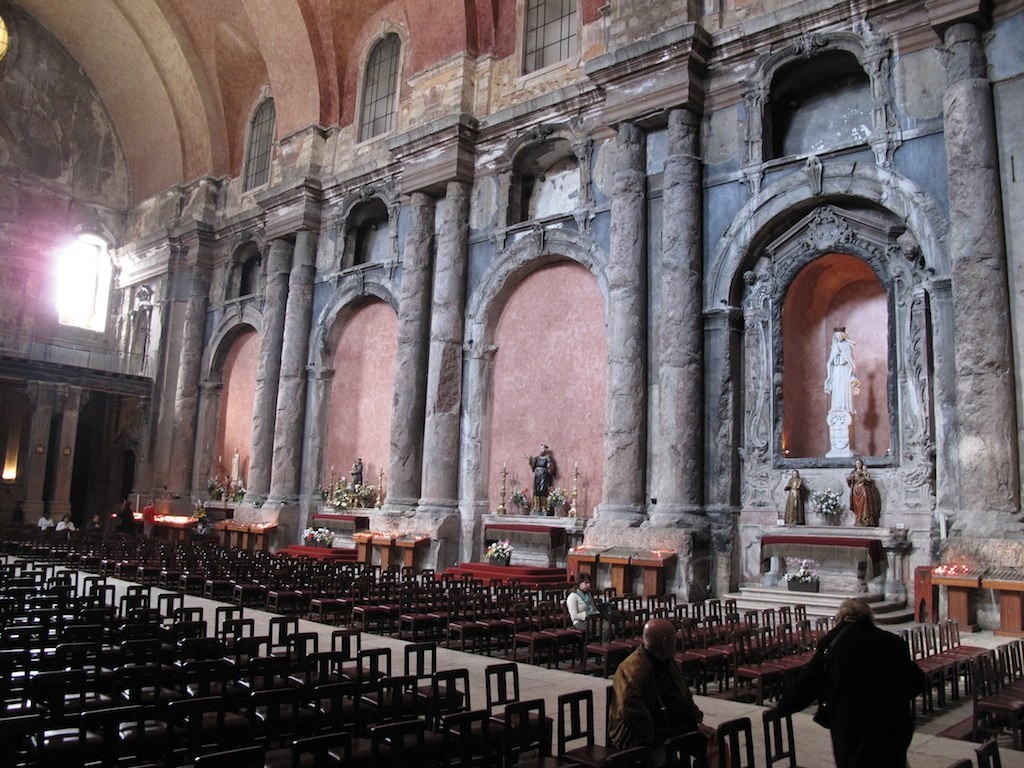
top-left (783, 470), bottom-right (805, 524)
top-left (221, 475), bottom-right (229, 497)
top-left (87, 513), bottom-right (104, 540)
top-left (143, 505), bottom-right (159, 537)
top-left (13, 501), bottom-right (24, 523)
top-left (55, 515), bottom-right (75, 538)
top-left (349, 458), bottom-right (363, 486)
top-left (525, 444), bottom-right (555, 512)
top-left (846, 459), bottom-right (880, 527)
top-left (798, 599), bottom-right (928, 768)
top-left (608, 618), bottom-right (732, 768)
top-left (566, 574), bottom-right (617, 643)
top-left (232, 448), bottom-right (240, 482)
top-left (824, 327), bottom-right (860, 415)
top-left (38, 511), bottom-right (53, 530)
top-left (114, 497), bottom-right (139, 533)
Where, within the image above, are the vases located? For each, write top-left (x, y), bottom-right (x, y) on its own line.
top-left (786, 578), bottom-right (819, 592)
top-left (304, 538), bottom-right (316, 547)
top-left (556, 505), bottom-right (569, 517)
top-left (361, 497), bottom-right (371, 508)
top-left (822, 512), bottom-right (839, 525)
top-left (520, 502), bottom-right (531, 515)
top-left (489, 556), bottom-right (510, 566)
top-left (317, 539), bottom-right (332, 548)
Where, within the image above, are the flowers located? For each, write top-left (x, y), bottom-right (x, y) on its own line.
top-left (485, 537), bottom-right (513, 560)
top-left (810, 487), bottom-right (846, 515)
top-left (354, 483), bottom-right (377, 500)
top-left (301, 526), bottom-right (339, 545)
top-left (192, 500), bottom-right (210, 526)
top-left (546, 486), bottom-right (574, 509)
top-left (784, 555), bottom-right (821, 582)
top-left (509, 485), bottom-right (528, 508)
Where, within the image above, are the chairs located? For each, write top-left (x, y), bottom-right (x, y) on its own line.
top-left (1, 524), bottom-right (1024, 768)
